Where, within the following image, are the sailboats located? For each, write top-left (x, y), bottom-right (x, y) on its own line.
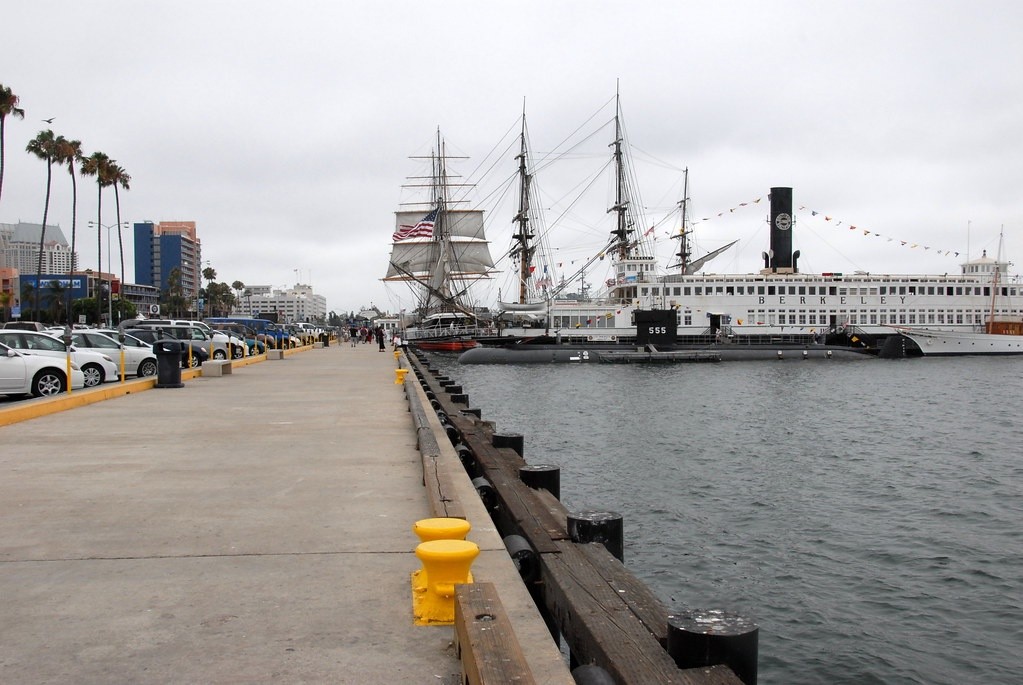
top-left (381, 75), bottom-right (1023, 355)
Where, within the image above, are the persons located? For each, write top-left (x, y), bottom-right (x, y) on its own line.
top-left (450, 321), bottom-right (455, 336)
top-left (336, 324), bottom-right (402, 352)
top-left (485, 319), bottom-right (495, 336)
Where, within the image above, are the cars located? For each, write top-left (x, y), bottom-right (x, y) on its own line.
top-left (0, 313), bottom-right (335, 399)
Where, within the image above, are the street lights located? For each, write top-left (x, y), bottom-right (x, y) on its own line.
top-left (87, 221), bottom-right (129, 331)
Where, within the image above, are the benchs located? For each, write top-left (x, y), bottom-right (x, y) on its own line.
top-left (314, 342), bottom-right (324, 348)
top-left (267, 348), bottom-right (284, 360)
top-left (202, 360), bottom-right (233, 376)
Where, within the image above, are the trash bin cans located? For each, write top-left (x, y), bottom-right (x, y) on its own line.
top-left (322, 333), bottom-right (330, 347)
top-left (153, 339), bottom-right (185, 387)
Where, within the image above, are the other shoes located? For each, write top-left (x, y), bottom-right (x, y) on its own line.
top-left (379, 350), bottom-right (381, 352)
top-left (381, 350), bottom-right (385, 352)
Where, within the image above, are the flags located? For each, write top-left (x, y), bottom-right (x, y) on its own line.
top-left (392, 206), bottom-right (439, 241)
top-left (575, 298), bottom-right (640, 330)
